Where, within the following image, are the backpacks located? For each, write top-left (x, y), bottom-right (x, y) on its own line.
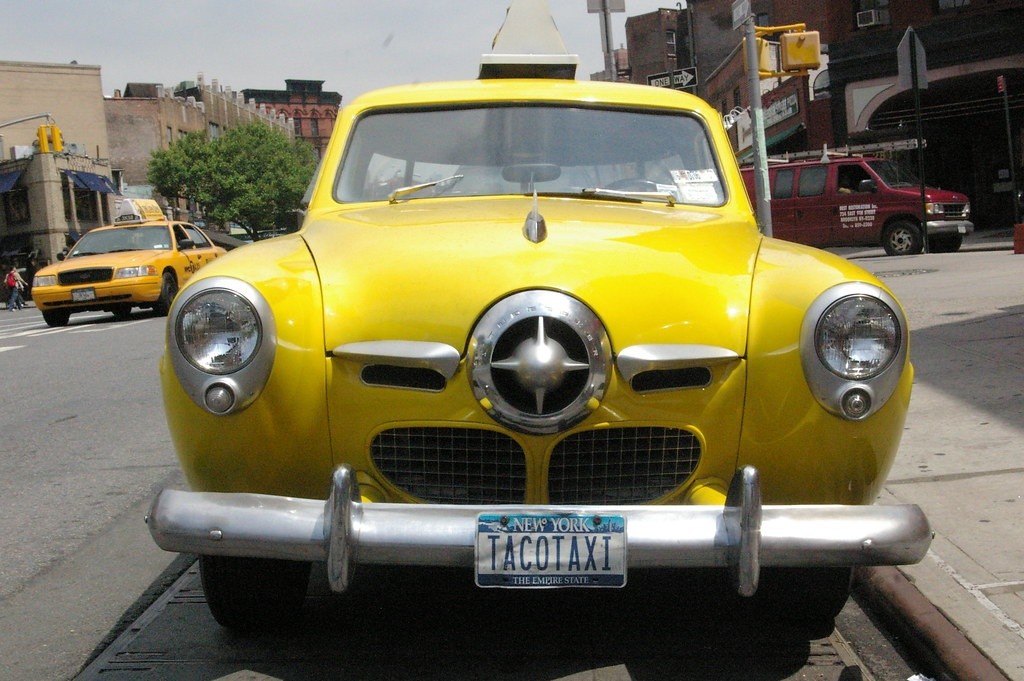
top-left (6, 271), bottom-right (17, 287)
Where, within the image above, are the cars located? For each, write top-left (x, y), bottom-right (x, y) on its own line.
top-left (27, 199), bottom-right (227, 329)
top-left (141, 1), bottom-right (937, 639)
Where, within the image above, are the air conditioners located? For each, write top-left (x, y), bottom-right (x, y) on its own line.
top-left (857, 9), bottom-right (881, 27)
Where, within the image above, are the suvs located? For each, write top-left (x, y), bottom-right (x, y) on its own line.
top-left (729, 149), bottom-right (977, 258)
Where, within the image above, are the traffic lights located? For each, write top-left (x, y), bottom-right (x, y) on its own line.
top-left (52, 125), bottom-right (65, 153)
top-left (32, 125), bottom-right (47, 154)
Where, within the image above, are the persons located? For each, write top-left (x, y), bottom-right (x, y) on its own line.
top-left (0, 259), bottom-right (42, 312)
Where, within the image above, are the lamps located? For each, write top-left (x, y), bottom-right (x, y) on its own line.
top-left (723, 105), bottom-right (745, 131)
